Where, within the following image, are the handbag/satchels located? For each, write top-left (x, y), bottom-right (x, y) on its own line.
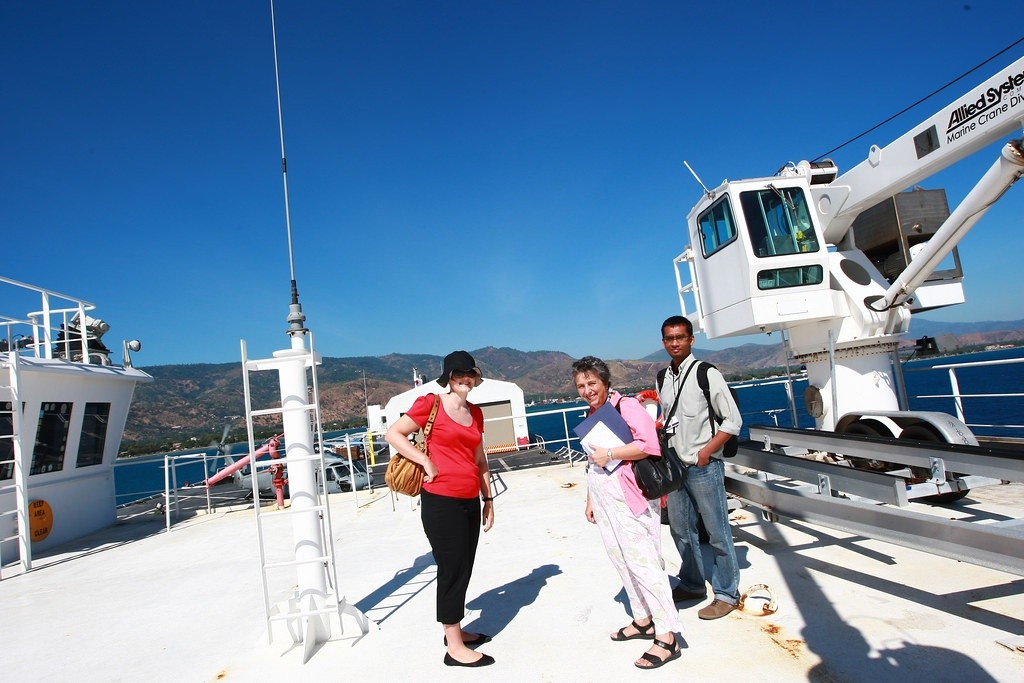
top-left (616, 395), bottom-right (688, 501)
top-left (385, 391), bottom-right (440, 497)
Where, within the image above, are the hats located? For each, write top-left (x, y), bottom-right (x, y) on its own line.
top-left (436, 350), bottom-right (483, 388)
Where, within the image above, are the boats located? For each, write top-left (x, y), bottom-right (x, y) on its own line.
top-left (0, 275), bottom-right (155, 567)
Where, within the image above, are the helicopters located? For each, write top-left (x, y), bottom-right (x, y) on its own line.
top-left (157, 423), bottom-right (389, 502)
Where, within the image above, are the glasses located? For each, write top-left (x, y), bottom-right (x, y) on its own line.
top-left (452, 369), bottom-right (477, 378)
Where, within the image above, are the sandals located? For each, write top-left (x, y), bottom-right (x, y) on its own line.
top-left (611, 621), bottom-right (656, 641)
top-left (634, 636), bottom-right (681, 668)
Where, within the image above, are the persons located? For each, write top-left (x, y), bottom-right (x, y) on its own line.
top-left (655, 316), bottom-right (742, 620)
top-left (571, 356), bottom-right (682, 669)
top-left (385, 351), bottom-right (495, 667)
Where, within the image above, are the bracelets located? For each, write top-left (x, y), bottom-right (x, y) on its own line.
top-left (483, 496), bottom-right (494, 502)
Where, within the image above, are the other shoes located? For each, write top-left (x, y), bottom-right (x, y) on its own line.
top-left (698, 599), bottom-right (738, 620)
top-left (443, 631), bottom-right (492, 646)
top-left (444, 651), bottom-right (495, 668)
top-left (671, 586), bottom-right (706, 602)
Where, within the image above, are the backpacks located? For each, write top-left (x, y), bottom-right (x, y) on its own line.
top-left (657, 362), bottom-right (741, 457)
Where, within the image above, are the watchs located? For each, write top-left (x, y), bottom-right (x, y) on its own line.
top-left (607, 448), bottom-right (614, 462)
